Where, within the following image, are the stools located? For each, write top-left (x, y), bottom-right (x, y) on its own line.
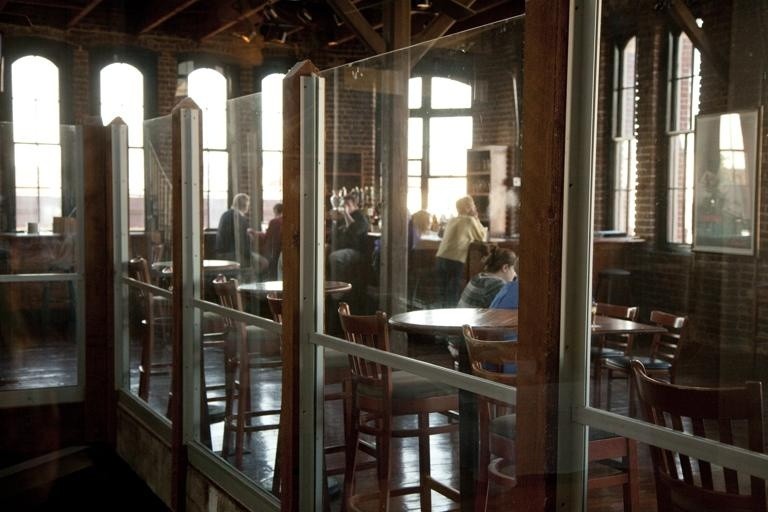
top-left (41, 259), bottom-right (77, 329)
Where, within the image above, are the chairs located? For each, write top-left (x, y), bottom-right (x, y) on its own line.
top-left (204, 274), bottom-right (284, 470)
top-left (605, 309), bottom-right (688, 412)
top-left (630, 358), bottom-right (768, 510)
top-left (131, 254), bottom-right (173, 402)
top-left (338, 301), bottom-right (476, 512)
top-left (462, 323), bottom-right (640, 511)
top-left (591, 301), bottom-right (642, 408)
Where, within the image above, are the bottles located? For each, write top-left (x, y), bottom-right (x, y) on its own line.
top-left (330, 186), bottom-right (375, 211)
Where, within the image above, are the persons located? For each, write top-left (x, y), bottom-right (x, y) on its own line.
top-left (478, 276), bottom-right (518, 374)
top-left (448, 242), bottom-right (519, 346)
top-left (251, 203), bottom-right (283, 273)
top-left (365, 201), bottom-right (431, 285)
top-left (329, 195), bottom-right (373, 279)
top-left (432, 194), bottom-right (487, 346)
top-left (214, 191), bottom-right (270, 274)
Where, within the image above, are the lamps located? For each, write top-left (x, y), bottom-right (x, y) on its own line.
top-left (240, 5), bottom-right (345, 45)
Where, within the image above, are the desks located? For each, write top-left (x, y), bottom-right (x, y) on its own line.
top-left (389, 306), bottom-right (668, 480)
top-left (150, 258), bottom-right (242, 275)
top-left (238, 280), bottom-right (353, 294)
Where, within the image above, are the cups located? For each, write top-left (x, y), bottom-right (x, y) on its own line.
top-left (28, 222), bottom-right (38, 234)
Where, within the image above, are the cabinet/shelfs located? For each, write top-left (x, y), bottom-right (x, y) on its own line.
top-left (465, 144), bottom-right (508, 235)
top-left (324, 149), bottom-right (365, 220)
